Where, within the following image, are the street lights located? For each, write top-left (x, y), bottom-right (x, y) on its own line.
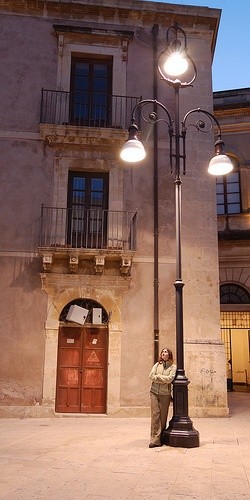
top-left (119, 18), bottom-right (233, 451)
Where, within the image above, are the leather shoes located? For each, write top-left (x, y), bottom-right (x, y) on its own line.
top-left (149, 443), bottom-right (162, 447)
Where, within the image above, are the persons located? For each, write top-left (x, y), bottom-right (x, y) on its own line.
top-left (149, 348), bottom-right (177, 448)
